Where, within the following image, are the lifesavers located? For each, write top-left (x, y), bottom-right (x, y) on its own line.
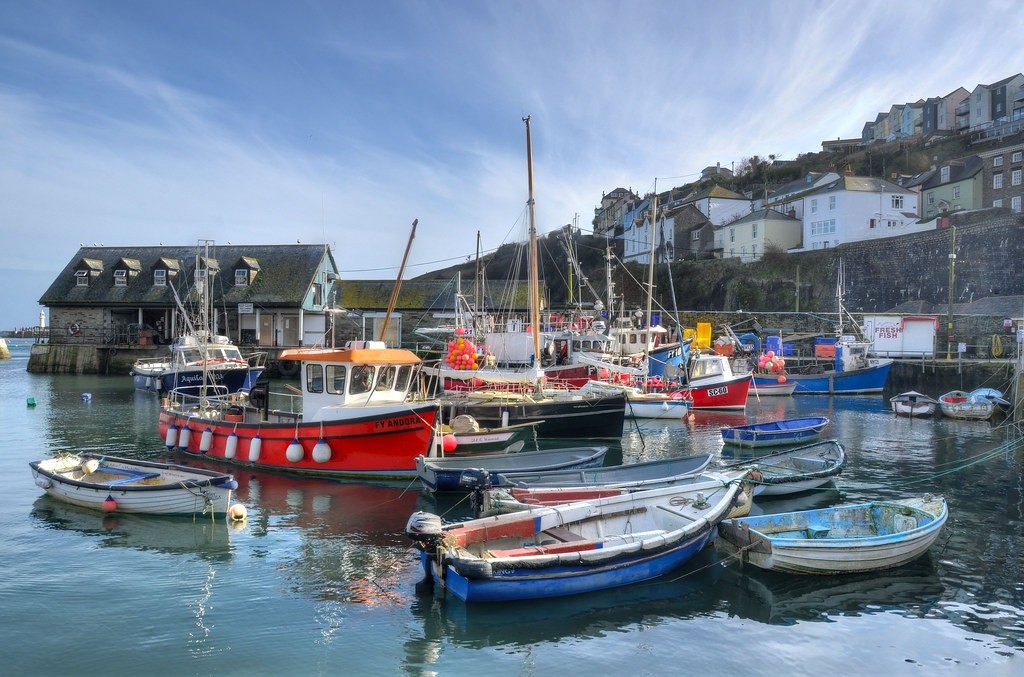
top-left (68, 323), bottom-right (80, 335)
top-left (684, 329), bottom-right (697, 348)
top-left (740, 332), bottom-right (759, 352)
top-left (108, 348), bottom-right (117, 356)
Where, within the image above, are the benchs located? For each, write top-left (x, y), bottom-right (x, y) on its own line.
top-left (94, 466), bottom-right (160, 486)
top-left (655, 504), bottom-right (699, 529)
top-left (792, 456), bottom-right (835, 471)
top-left (543, 528), bottom-right (588, 542)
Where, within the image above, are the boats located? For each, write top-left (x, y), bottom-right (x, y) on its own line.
top-left (939, 390), bottom-right (995, 420)
top-left (721, 417), bottom-right (830, 448)
top-left (459, 468), bottom-right (651, 519)
top-left (719, 439), bottom-right (847, 495)
top-left (497, 453), bottom-right (714, 488)
top-left (714, 492), bottom-right (950, 574)
top-left (413, 446), bottom-right (610, 492)
top-left (129, 240), bottom-right (268, 398)
top-left (971, 388), bottom-right (1012, 414)
top-left (437, 414), bottom-right (545, 458)
top-left (29, 450), bottom-right (239, 517)
top-left (158, 340), bottom-right (441, 477)
top-left (889, 390), bottom-right (939, 419)
top-left (749, 383), bottom-right (797, 395)
top-left (406, 464), bottom-right (758, 603)
top-left (747, 257), bottom-right (894, 395)
top-left (377, 114), bottom-right (754, 441)
